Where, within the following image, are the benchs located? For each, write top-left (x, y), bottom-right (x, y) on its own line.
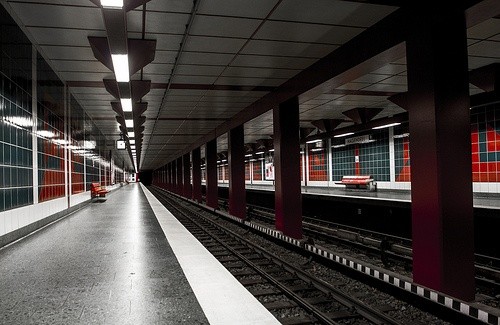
top-left (90, 182), bottom-right (110, 200)
top-left (335, 175), bottom-right (377, 193)
